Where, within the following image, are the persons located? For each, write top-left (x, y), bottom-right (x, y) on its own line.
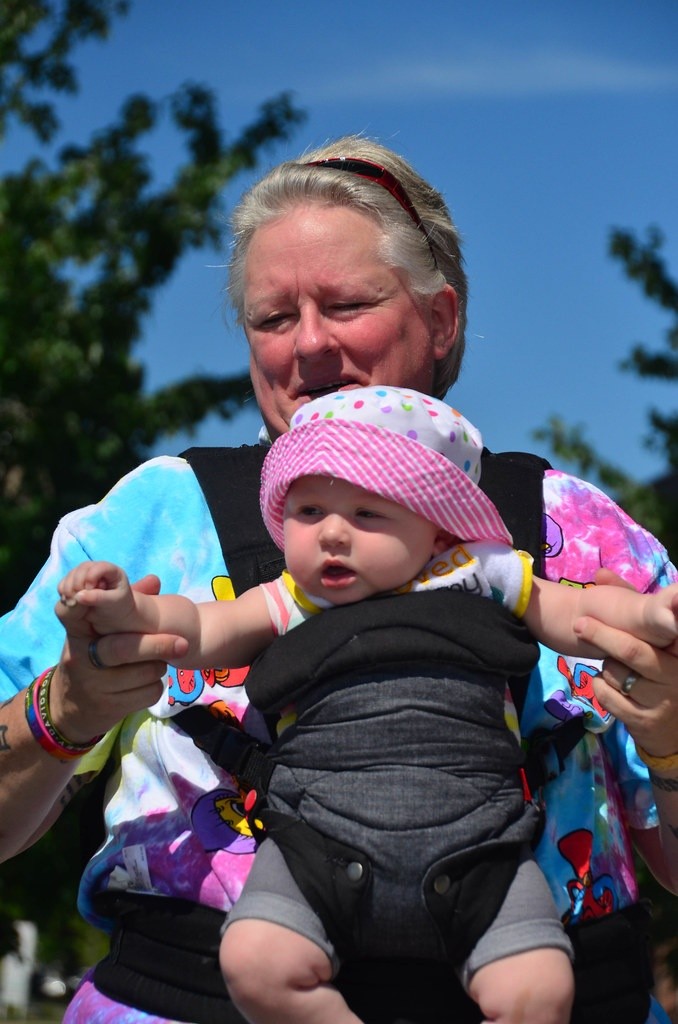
top-left (0, 133), bottom-right (677, 1023)
top-left (48, 385), bottom-right (677, 1023)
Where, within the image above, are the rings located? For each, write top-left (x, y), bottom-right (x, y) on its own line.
top-left (86, 632), bottom-right (110, 671)
top-left (620, 671), bottom-right (639, 699)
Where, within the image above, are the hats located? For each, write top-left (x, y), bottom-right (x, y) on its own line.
top-left (258, 384), bottom-right (514, 554)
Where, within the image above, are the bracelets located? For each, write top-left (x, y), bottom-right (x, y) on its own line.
top-left (646, 768), bottom-right (678, 797)
top-left (40, 664), bottom-right (107, 751)
top-left (632, 742), bottom-right (678, 774)
top-left (22, 664), bottom-right (89, 759)
